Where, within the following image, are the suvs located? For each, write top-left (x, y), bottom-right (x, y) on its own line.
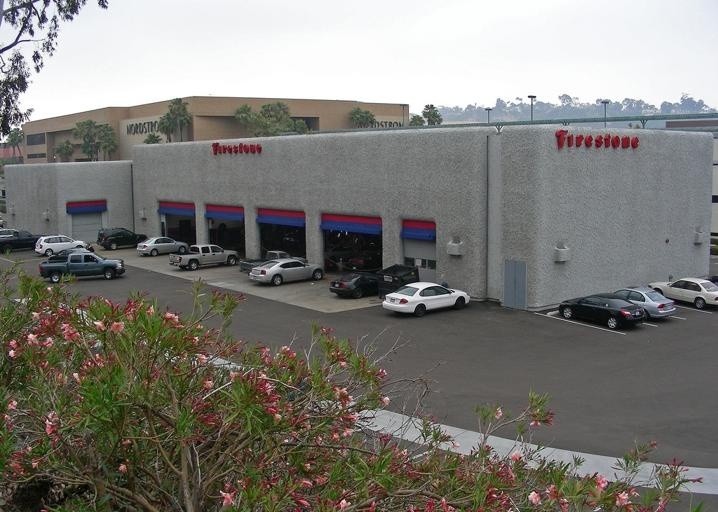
top-left (35, 235), bottom-right (93, 255)
top-left (97, 228), bottom-right (147, 250)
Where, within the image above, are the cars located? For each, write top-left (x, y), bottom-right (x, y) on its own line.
top-left (249, 258), bottom-right (325, 285)
top-left (649, 277), bottom-right (718, 309)
top-left (0, 228), bottom-right (40, 253)
top-left (559, 294), bottom-right (646, 329)
top-left (383, 281), bottom-right (473, 318)
top-left (137, 236), bottom-right (189, 257)
top-left (329, 271), bottom-right (378, 298)
top-left (613, 285), bottom-right (675, 318)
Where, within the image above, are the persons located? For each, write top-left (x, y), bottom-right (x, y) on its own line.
top-left (86, 243), bottom-right (94, 252)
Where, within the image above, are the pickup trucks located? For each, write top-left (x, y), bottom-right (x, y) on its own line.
top-left (39, 248), bottom-right (126, 283)
top-left (237, 251), bottom-right (290, 275)
top-left (169, 244), bottom-right (238, 271)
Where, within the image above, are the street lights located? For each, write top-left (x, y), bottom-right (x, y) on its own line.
top-left (601, 101), bottom-right (609, 126)
top-left (486, 108), bottom-right (492, 122)
top-left (527, 95), bottom-right (536, 120)
